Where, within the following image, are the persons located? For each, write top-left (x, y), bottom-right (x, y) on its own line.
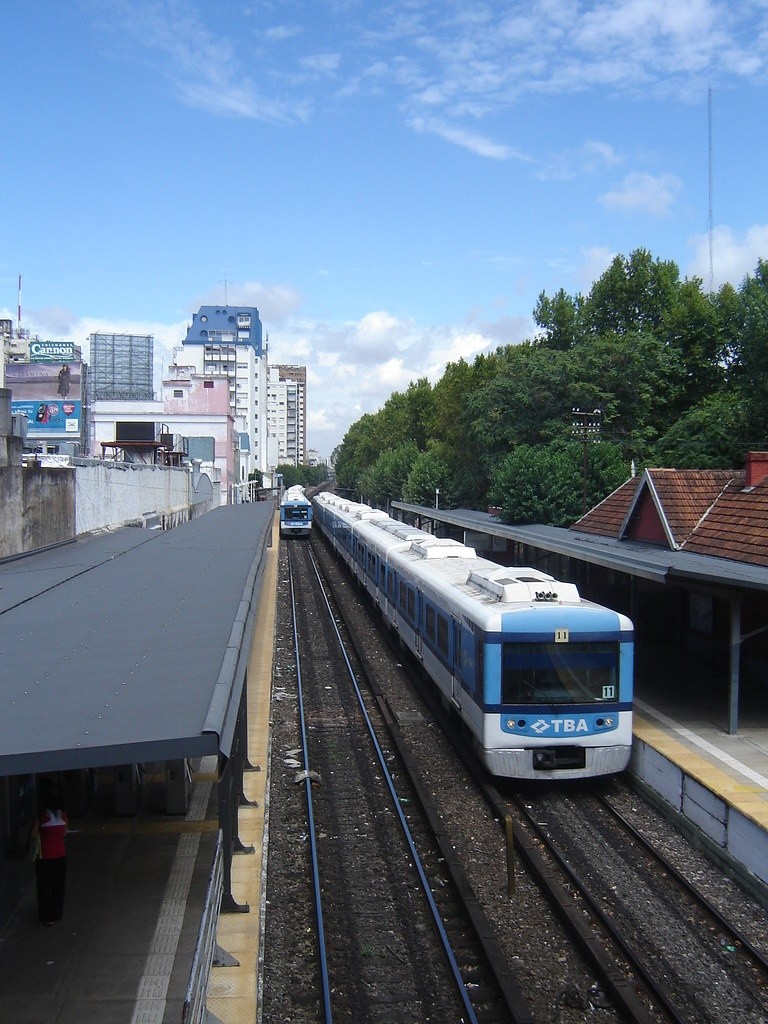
top-left (57, 363), bottom-right (72, 400)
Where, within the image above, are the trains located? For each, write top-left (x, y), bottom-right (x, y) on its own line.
top-left (310, 488), bottom-right (637, 791)
top-left (279, 484), bottom-right (314, 536)
